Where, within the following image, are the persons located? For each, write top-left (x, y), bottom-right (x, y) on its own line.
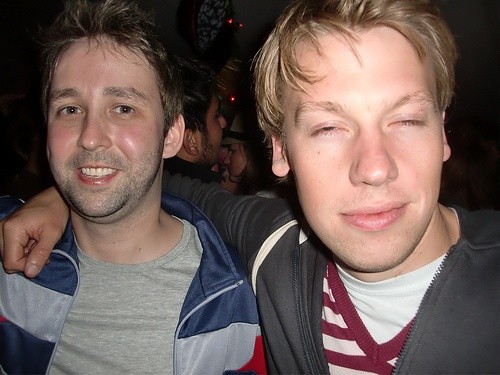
top-left (163, 52), bottom-right (277, 197)
top-left (1, 1), bottom-right (271, 375)
top-left (0, 0), bottom-right (500, 375)
